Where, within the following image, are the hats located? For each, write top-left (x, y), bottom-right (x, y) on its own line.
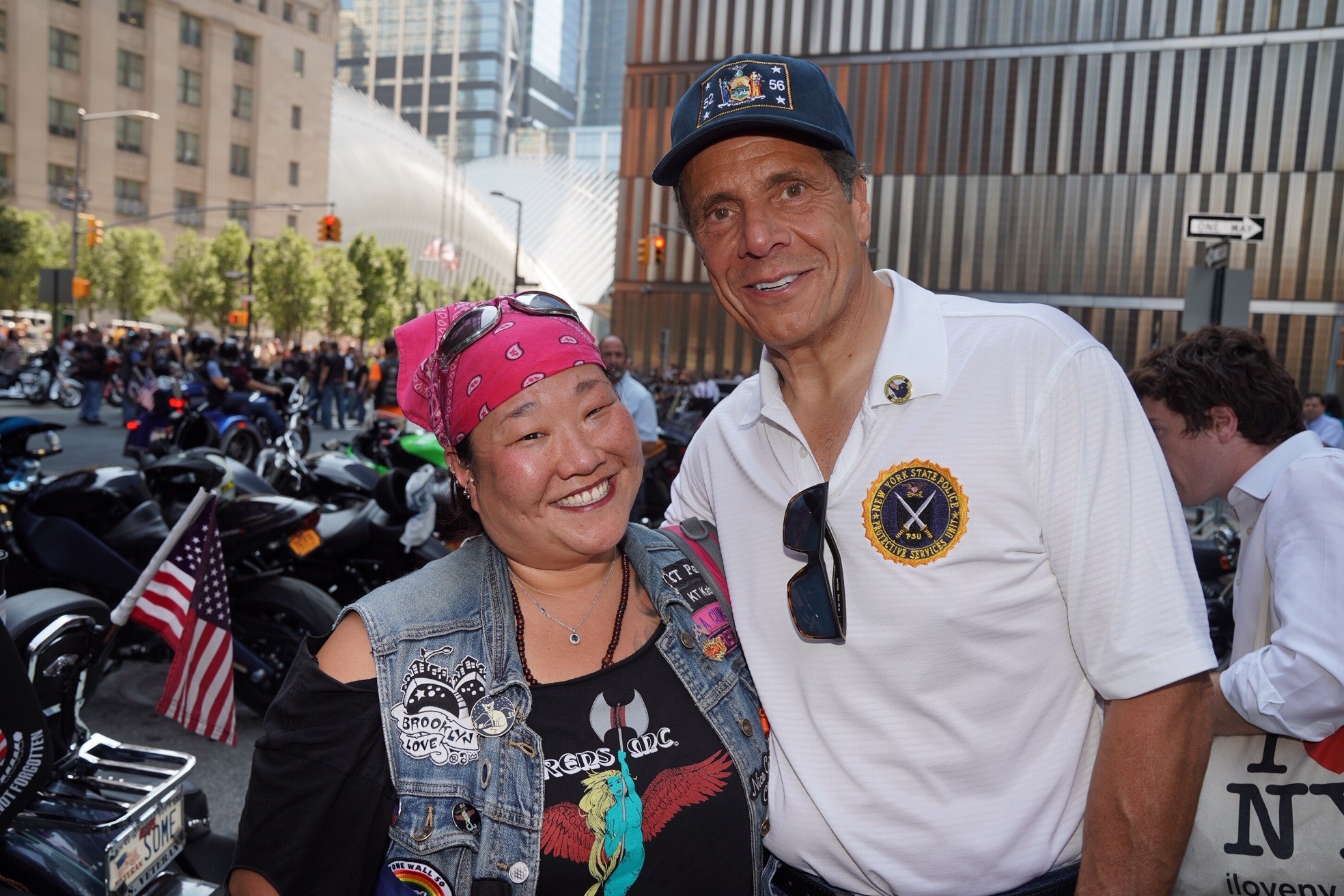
top-left (650, 53), bottom-right (854, 186)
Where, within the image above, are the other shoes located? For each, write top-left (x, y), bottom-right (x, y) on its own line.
top-left (82, 418), bottom-right (101, 426)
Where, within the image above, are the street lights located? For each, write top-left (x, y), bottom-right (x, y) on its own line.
top-left (489, 190), bottom-right (522, 297)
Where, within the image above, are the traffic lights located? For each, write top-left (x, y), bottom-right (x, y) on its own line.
top-left (76, 214), bottom-right (103, 248)
top-left (71, 278), bottom-right (95, 299)
top-left (655, 236), bottom-right (665, 265)
top-left (318, 218), bottom-right (328, 242)
top-left (228, 311), bottom-right (247, 327)
top-left (325, 216), bottom-right (335, 240)
top-left (637, 235), bottom-right (651, 266)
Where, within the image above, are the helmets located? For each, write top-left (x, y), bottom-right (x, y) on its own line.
top-left (193, 334), bottom-right (219, 358)
top-left (218, 339), bottom-right (240, 361)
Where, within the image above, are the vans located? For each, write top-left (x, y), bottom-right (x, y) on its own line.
top-left (3, 309), bottom-right (52, 329)
top-left (104, 320), bottom-right (179, 338)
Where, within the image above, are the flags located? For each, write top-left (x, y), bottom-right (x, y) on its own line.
top-left (111, 486), bottom-right (238, 748)
top-left (424, 239), bottom-right (458, 271)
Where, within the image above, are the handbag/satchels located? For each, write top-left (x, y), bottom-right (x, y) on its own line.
top-left (1191, 563), bottom-right (1344, 896)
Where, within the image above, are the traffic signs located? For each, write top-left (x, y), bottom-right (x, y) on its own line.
top-left (1181, 211), bottom-right (1268, 246)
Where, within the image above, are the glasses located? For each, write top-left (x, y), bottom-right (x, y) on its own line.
top-left (782, 480), bottom-right (847, 646)
top-left (437, 291), bottom-right (582, 444)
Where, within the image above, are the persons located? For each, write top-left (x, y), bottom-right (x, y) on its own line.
top-left (1124, 323), bottom-right (1344, 741)
top-left (0, 317), bottom-right (402, 459)
top-left (651, 55), bottom-right (1217, 896)
top-left (223, 290), bottom-right (771, 896)
top-left (597, 335), bottom-right (748, 525)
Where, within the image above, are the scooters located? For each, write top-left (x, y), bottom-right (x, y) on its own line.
top-left (0, 320), bottom-right (477, 719)
top-left (0, 584), bottom-right (215, 895)
top-left (1189, 509), bottom-right (1242, 660)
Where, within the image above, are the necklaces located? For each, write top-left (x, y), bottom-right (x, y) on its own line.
top-left (506, 546), bottom-right (629, 684)
